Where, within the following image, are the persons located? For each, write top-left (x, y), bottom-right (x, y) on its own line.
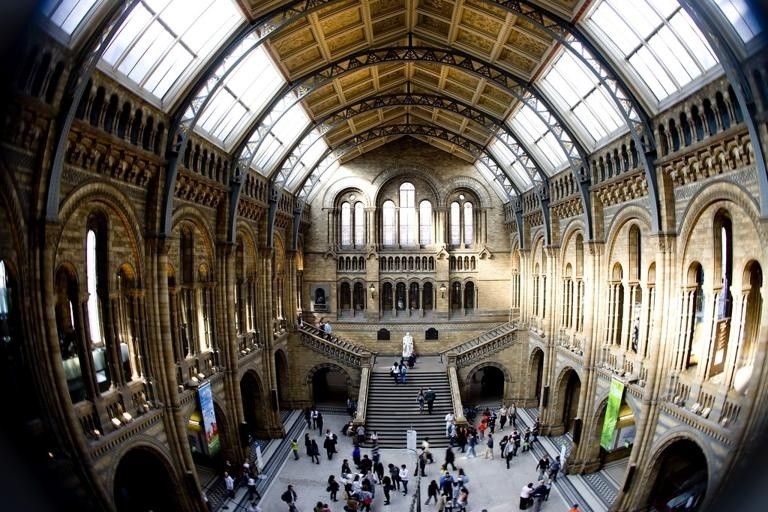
top-left (566, 504), bottom-right (582, 512)
top-left (324, 321), bottom-right (332, 342)
top-left (402, 331), bottom-right (414, 358)
top-left (389, 347), bottom-right (416, 384)
top-left (297, 312), bottom-right (305, 328)
top-left (279, 387), bottom-right (561, 512)
top-left (318, 317), bottom-right (325, 339)
top-left (221, 454), bottom-right (263, 512)
